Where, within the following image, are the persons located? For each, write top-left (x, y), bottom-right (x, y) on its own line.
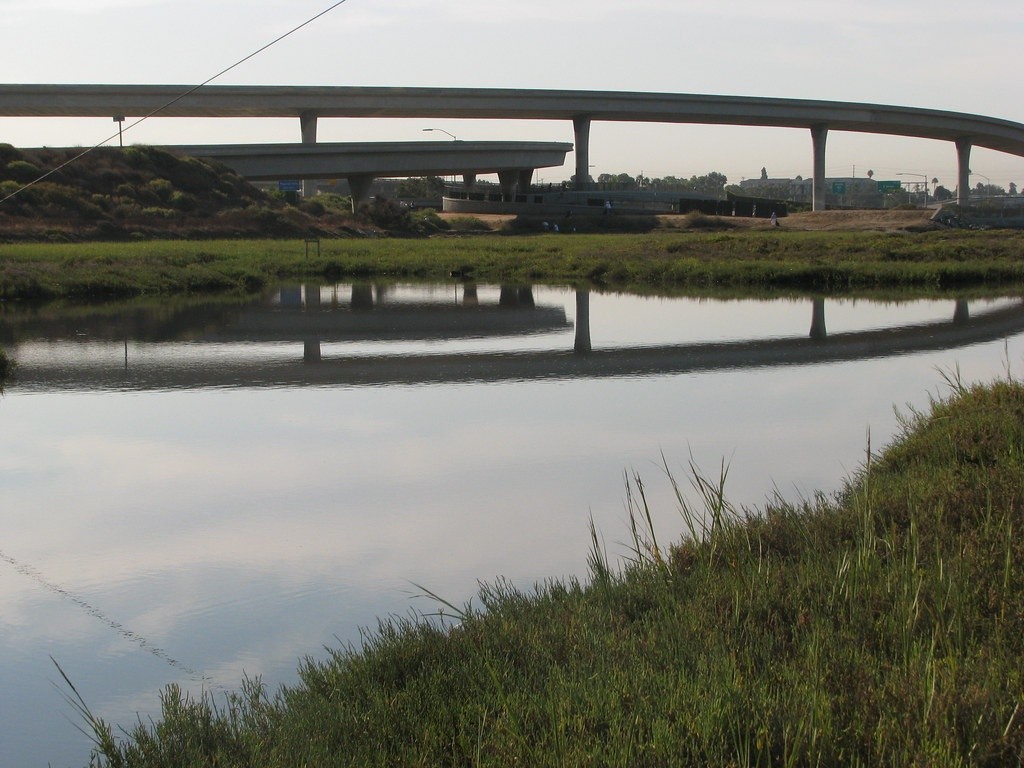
top-left (770, 211), bottom-right (777, 227)
top-left (604, 200), bottom-right (614, 217)
top-left (542, 221), bottom-right (549, 232)
top-left (550, 223), bottom-right (559, 233)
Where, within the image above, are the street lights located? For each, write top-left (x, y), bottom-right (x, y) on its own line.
top-left (421, 127), bottom-right (457, 182)
top-left (896, 173), bottom-right (928, 208)
top-left (968, 173), bottom-right (990, 197)
